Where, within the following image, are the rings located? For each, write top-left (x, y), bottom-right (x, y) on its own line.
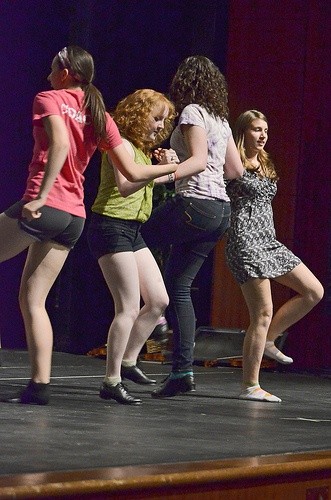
top-left (171, 157), bottom-right (173, 160)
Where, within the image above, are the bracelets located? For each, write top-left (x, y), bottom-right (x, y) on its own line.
top-left (168, 173), bottom-right (174, 183)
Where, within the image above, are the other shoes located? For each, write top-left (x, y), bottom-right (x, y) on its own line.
top-left (0, 378), bottom-right (51, 405)
top-left (151, 373), bottom-right (198, 397)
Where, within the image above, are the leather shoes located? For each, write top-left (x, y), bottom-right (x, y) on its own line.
top-left (99, 381), bottom-right (143, 405)
top-left (120, 364), bottom-right (158, 386)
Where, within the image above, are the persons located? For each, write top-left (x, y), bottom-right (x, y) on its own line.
top-left (225, 110), bottom-right (324, 402)
top-left (86, 89), bottom-right (180, 407)
top-left (0, 46), bottom-right (178, 406)
top-left (140, 56), bottom-right (244, 398)
